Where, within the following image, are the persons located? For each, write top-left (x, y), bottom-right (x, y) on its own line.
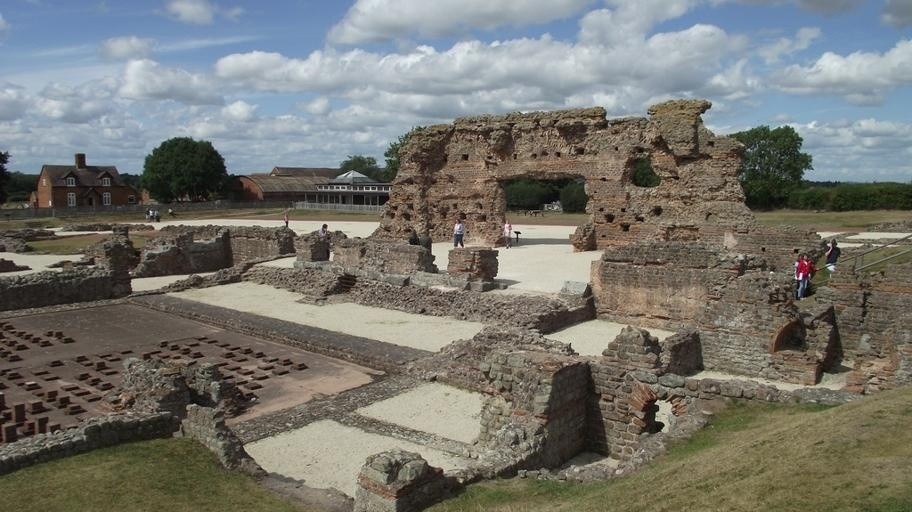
top-left (319, 224), bottom-right (328, 241)
top-left (824, 238), bottom-right (847, 279)
top-left (284, 214), bottom-right (288, 227)
top-left (794, 253), bottom-right (819, 301)
top-left (168, 207), bottom-right (176, 215)
top-left (145, 207), bottom-right (161, 222)
top-left (504, 220), bottom-right (512, 249)
top-left (453, 219), bottom-right (464, 248)
